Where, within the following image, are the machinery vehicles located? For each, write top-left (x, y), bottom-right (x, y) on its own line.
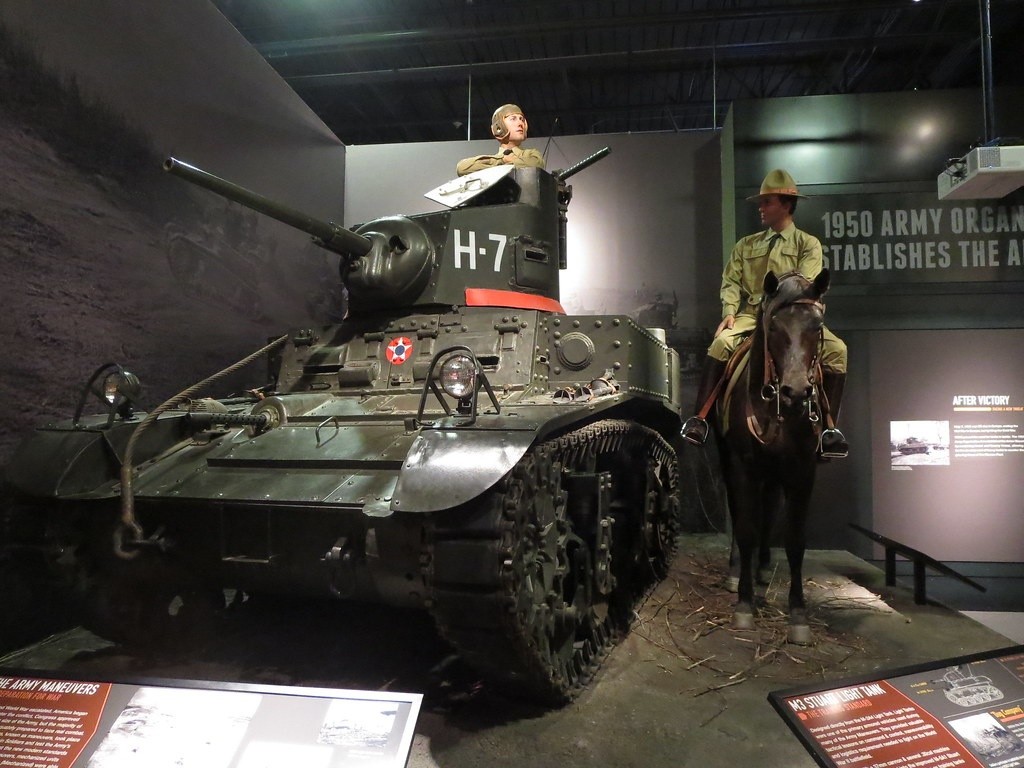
top-left (5, 146), bottom-right (684, 706)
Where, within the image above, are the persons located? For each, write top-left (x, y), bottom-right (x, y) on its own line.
top-left (454, 104), bottom-right (545, 172)
top-left (685, 169), bottom-right (849, 455)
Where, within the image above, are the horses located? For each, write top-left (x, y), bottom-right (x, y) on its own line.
top-left (692, 266), bottom-right (833, 649)
